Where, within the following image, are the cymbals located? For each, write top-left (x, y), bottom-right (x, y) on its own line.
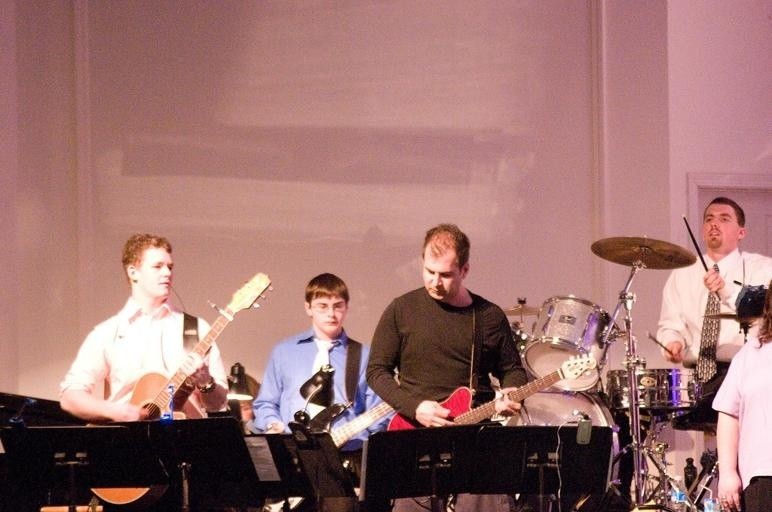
top-left (705, 314), bottom-right (767, 323)
top-left (504, 306), bottom-right (540, 318)
top-left (589, 237), bottom-right (696, 269)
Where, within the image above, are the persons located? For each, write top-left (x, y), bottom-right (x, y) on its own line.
top-left (59, 234), bottom-right (231, 511)
top-left (645, 196), bottom-right (771, 376)
top-left (710, 289), bottom-right (772, 511)
top-left (364, 224), bottom-right (525, 511)
top-left (251, 273), bottom-right (399, 511)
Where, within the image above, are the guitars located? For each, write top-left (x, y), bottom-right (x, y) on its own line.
top-left (388, 353), bottom-right (597, 431)
top-left (265, 400), bottom-right (395, 512)
top-left (88, 273), bottom-right (274, 508)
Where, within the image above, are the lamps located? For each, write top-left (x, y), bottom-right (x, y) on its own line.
top-left (294, 364), bottom-right (334, 424)
top-left (226, 362), bottom-right (261, 434)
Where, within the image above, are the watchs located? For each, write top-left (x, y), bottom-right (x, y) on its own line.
top-left (197, 376), bottom-right (216, 393)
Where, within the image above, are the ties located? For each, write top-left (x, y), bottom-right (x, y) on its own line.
top-left (697, 264), bottom-right (721, 383)
top-left (312, 338), bottom-right (340, 376)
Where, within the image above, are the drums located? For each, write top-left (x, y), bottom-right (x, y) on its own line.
top-left (496, 390), bottom-right (622, 512)
top-left (520, 296), bottom-right (621, 390)
top-left (607, 371), bottom-right (698, 413)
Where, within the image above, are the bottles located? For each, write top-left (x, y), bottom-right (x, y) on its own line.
top-left (671, 477), bottom-right (686, 512)
top-left (161, 384), bottom-right (174, 425)
